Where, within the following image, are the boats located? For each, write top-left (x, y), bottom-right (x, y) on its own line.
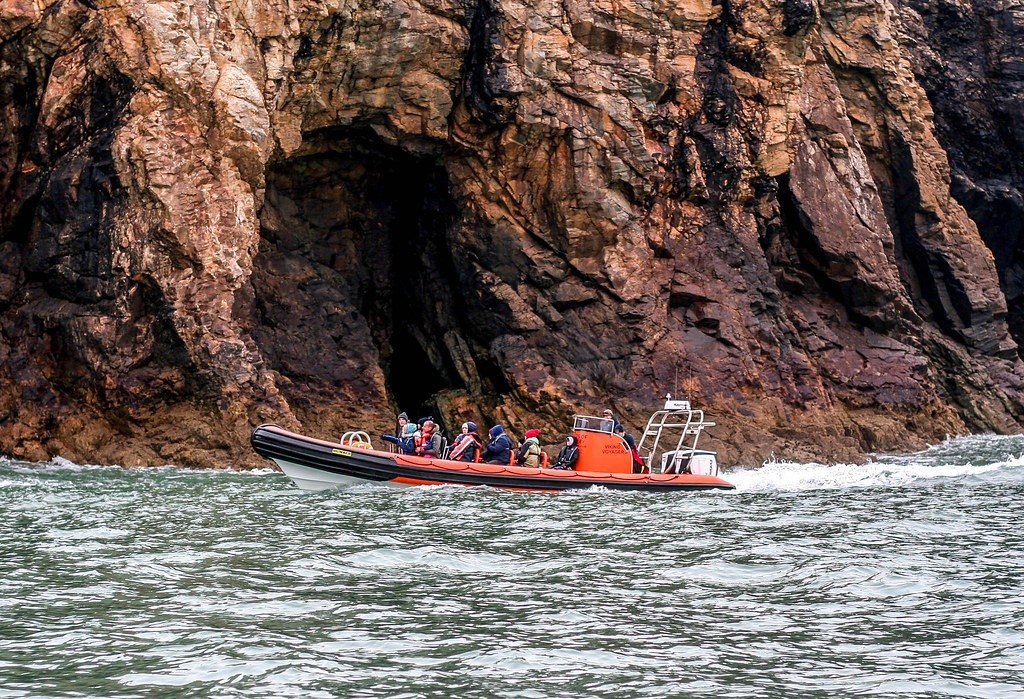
top-left (250, 366), bottom-right (736, 495)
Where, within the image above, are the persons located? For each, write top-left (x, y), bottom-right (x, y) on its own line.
top-left (398, 409), bottom-right (642, 473)
top-left (557, 436), bottom-right (578, 470)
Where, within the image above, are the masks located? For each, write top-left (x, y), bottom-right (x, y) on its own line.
top-left (567, 442), bottom-right (570, 446)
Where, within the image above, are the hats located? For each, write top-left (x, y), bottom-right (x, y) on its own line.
top-left (603, 409), bottom-right (613, 416)
top-left (467, 422), bottom-right (477, 431)
top-left (519, 437), bottom-right (525, 445)
top-left (398, 412), bottom-right (408, 422)
top-left (462, 423), bottom-right (468, 429)
top-left (418, 417), bottom-right (428, 426)
top-left (407, 423), bottom-right (417, 434)
top-left (525, 429), bottom-right (540, 438)
